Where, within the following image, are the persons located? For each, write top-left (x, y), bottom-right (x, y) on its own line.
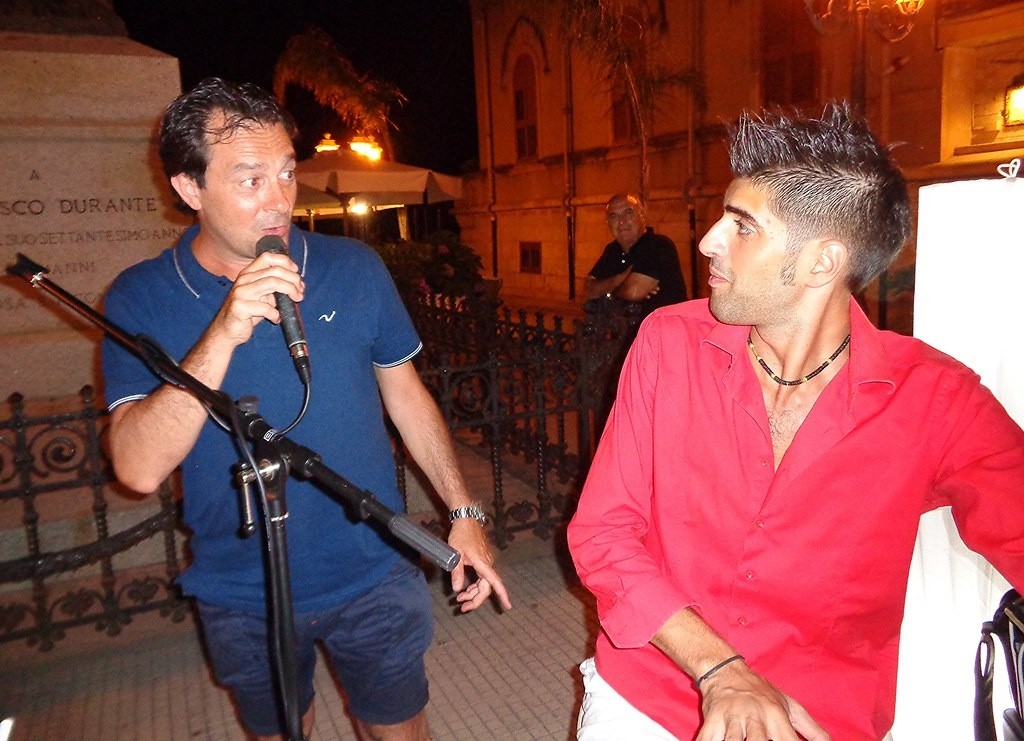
top-left (583, 193), bottom-right (686, 302)
top-left (103, 78), bottom-right (512, 741)
top-left (567, 113), bottom-right (1024, 741)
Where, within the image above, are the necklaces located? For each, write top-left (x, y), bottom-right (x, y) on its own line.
top-left (748, 334), bottom-right (850, 385)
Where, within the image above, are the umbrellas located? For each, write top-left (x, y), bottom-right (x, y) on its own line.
top-left (293, 149), bottom-right (462, 238)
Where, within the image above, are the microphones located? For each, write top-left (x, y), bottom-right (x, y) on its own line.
top-left (254, 235), bottom-right (313, 383)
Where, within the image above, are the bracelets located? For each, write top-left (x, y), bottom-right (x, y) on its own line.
top-left (696, 655), bottom-right (742, 687)
top-left (448, 507), bottom-right (488, 523)
